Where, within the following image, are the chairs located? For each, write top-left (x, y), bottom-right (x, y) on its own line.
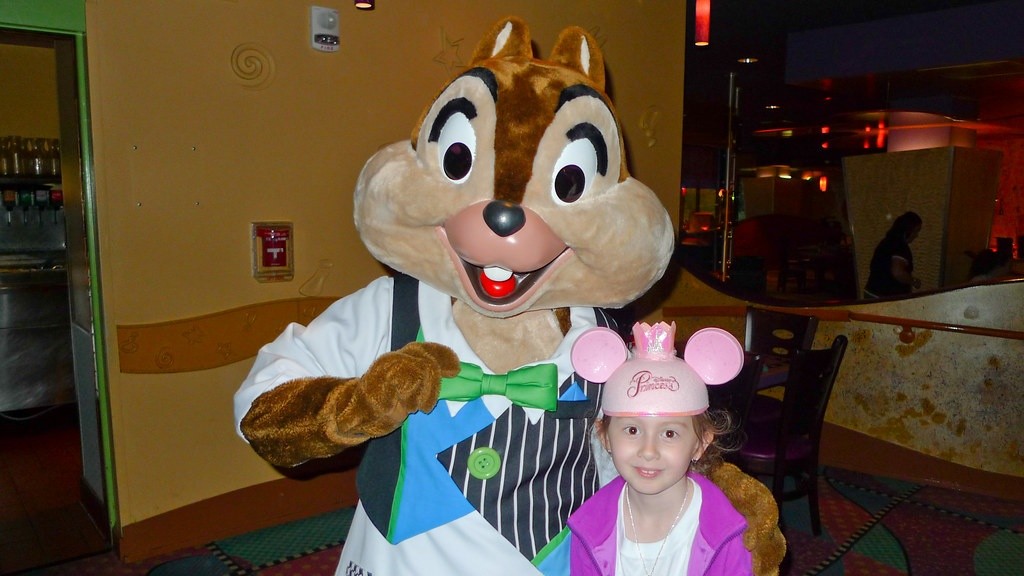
top-left (695, 306), bottom-right (848, 537)
top-left (778, 239), bottom-right (810, 289)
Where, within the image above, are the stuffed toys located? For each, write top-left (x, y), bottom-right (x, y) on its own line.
top-left (233, 17), bottom-right (789, 575)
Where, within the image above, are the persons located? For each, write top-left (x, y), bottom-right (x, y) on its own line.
top-left (861, 212), bottom-right (920, 299)
top-left (566, 318), bottom-right (754, 575)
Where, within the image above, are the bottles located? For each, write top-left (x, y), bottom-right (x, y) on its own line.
top-left (0, 135), bottom-right (64, 228)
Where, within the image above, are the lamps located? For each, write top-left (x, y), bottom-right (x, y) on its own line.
top-left (695, 0), bottom-right (710, 46)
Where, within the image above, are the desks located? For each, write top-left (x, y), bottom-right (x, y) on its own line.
top-left (673, 342), bottom-right (790, 389)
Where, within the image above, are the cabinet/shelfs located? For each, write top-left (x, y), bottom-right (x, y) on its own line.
top-left (1, 174), bottom-right (76, 409)
